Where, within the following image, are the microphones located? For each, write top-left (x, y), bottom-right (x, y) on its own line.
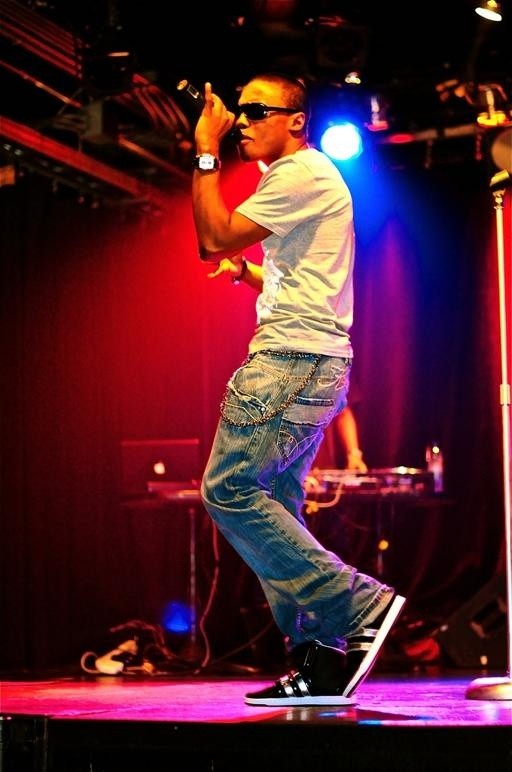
top-left (176, 78), bottom-right (242, 143)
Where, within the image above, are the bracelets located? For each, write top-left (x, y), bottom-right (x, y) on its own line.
top-left (230, 256), bottom-right (247, 285)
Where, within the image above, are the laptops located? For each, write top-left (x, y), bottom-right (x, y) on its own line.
top-left (116, 436), bottom-right (202, 499)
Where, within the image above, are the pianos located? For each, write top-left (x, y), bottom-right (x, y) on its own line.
top-left (161, 466), bottom-right (437, 508)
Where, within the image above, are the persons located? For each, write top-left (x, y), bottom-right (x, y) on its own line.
top-left (188, 70), bottom-right (410, 709)
top-left (214, 402), bottom-right (368, 674)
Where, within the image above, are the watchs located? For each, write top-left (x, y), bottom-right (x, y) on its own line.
top-left (191, 153), bottom-right (222, 173)
top-left (346, 449), bottom-right (363, 458)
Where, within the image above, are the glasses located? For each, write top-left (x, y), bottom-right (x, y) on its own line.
top-left (233, 102), bottom-right (297, 120)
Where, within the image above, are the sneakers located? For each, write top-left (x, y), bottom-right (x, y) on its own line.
top-left (244, 640), bottom-right (357, 707)
top-left (338, 591), bottom-right (408, 699)
